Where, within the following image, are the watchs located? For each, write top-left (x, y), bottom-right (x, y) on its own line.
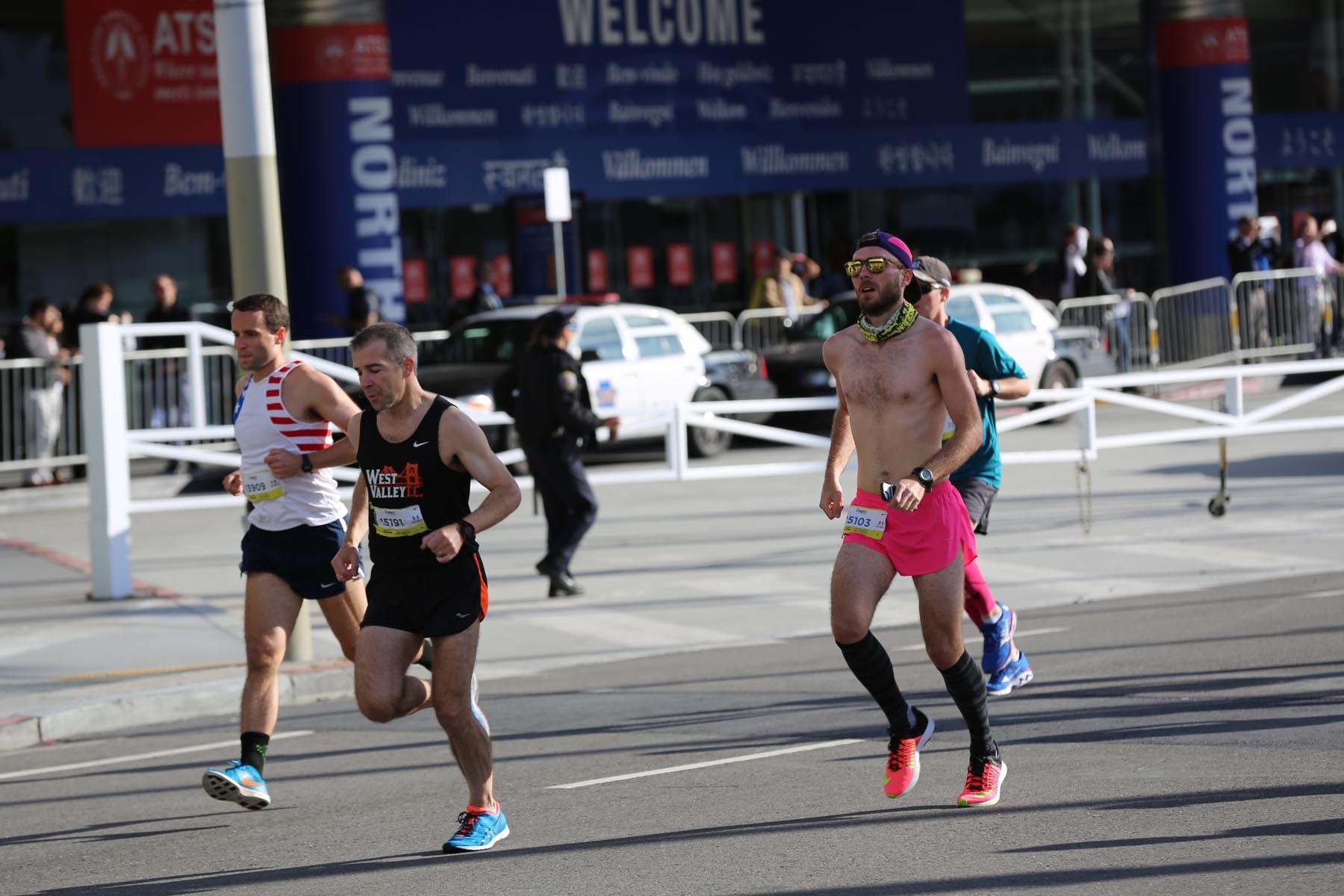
top-left (911, 467), bottom-right (933, 492)
top-left (985, 378), bottom-right (999, 401)
top-left (301, 453), bottom-right (312, 473)
top-left (456, 519), bottom-right (475, 544)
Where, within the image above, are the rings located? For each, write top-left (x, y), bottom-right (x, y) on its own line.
top-left (437, 551), bottom-right (442, 556)
top-left (335, 574), bottom-right (339, 578)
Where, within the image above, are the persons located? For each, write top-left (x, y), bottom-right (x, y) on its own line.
top-left (514, 305), bottom-right (620, 597)
top-left (1224, 215), bottom-right (1344, 365)
top-left (905, 255), bottom-right (1034, 697)
top-left (330, 325), bottom-right (522, 850)
top-left (747, 245), bottom-right (828, 345)
top-left (340, 266), bottom-right (380, 336)
top-left (0, 273), bottom-right (200, 487)
top-left (201, 293), bottom-right (362, 810)
top-left (819, 229), bottom-right (1007, 809)
top-left (1056, 223), bottom-right (1142, 395)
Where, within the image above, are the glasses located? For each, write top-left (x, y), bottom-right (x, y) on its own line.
top-left (845, 256), bottom-right (904, 277)
top-left (920, 282), bottom-right (944, 294)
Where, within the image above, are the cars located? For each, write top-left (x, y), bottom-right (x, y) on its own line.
top-left (755, 283), bottom-right (1110, 436)
top-left (330, 302), bottom-right (781, 473)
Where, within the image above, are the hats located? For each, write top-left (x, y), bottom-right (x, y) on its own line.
top-left (912, 255), bottom-right (951, 290)
top-left (850, 232), bottom-right (921, 305)
top-left (537, 305), bottom-right (576, 333)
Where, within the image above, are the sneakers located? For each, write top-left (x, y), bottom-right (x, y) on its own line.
top-left (980, 601), bottom-right (1017, 674)
top-left (442, 802), bottom-right (510, 854)
top-left (985, 651), bottom-right (1034, 695)
top-left (884, 706), bottom-right (934, 799)
top-left (202, 758), bottom-right (271, 810)
top-left (471, 674), bottom-right (490, 737)
top-left (957, 740), bottom-right (1007, 806)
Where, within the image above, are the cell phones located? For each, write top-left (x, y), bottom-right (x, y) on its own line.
top-left (880, 481), bottom-right (918, 511)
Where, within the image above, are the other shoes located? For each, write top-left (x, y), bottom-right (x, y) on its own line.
top-left (24, 471), bottom-right (52, 488)
top-left (537, 560), bottom-right (584, 597)
